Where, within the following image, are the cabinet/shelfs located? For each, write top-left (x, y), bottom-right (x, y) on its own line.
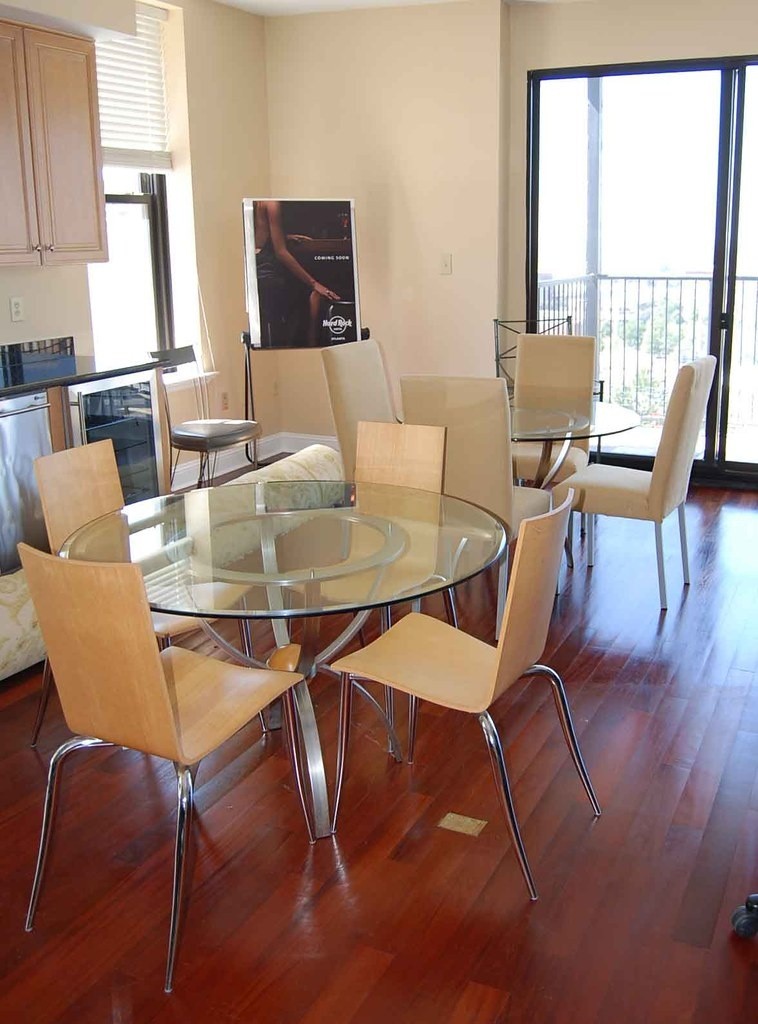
top-left (0, 16), bottom-right (108, 266)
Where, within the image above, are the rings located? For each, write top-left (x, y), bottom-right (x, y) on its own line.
top-left (326, 291), bottom-right (329, 293)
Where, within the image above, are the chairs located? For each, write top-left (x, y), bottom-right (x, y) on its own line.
top-left (319, 340), bottom-right (394, 558)
top-left (511, 334), bottom-right (598, 567)
top-left (557, 356), bottom-right (715, 616)
top-left (331, 487), bottom-right (595, 898)
top-left (148, 344), bottom-right (259, 489)
top-left (282, 416), bottom-right (448, 748)
top-left (29, 437), bottom-right (216, 772)
top-left (398, 376), bottom-right (554, 638)
top-left (12, 541), bottom-right (316, 995)
top-left (493, 317), bottom-right (605, 463)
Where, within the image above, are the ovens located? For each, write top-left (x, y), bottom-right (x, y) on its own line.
top-left (0, 388), bottom-right (54, 575)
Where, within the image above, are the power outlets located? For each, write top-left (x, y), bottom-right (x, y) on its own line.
top-left (10, 297), bottom-right (23, 322)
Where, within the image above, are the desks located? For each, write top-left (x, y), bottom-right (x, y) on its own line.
top-left (60, 478), bottom-right (506, 841)
top-left (395, 399), bottom-right (641, 489)
top-left (0, 358), bottom-right (178, 680)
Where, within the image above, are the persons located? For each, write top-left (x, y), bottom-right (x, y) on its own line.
top-left (254, 201), bottom-right (340, 347)
